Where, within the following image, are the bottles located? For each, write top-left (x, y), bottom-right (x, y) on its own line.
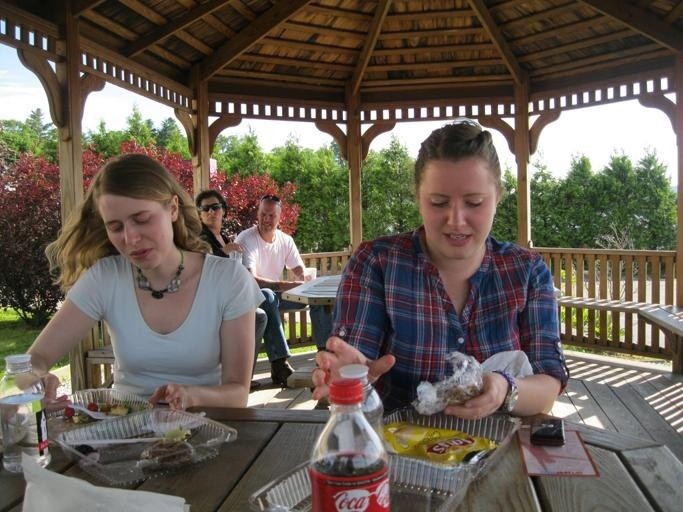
top-left (308, 363), bottom-right (388, 512)
top-left (0, 352), bottom-right (52, 474)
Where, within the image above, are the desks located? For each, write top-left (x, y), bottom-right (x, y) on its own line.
top-left (281, 272), bottom-right (351, 361)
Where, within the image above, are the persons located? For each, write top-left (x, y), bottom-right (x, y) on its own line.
top-left (234, 196), bottom-right (339, 386)
top-left (195, 189), bottom-right (268, 390)
top-left (311, 118), bottom-right (570, 424)
top-left (14, 151), bottom-right (267, 410)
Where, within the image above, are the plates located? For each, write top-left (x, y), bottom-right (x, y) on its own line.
top-left (246, 398), bottom-right (524, 511)
top-left (46, 387), bottom-right (236, 486)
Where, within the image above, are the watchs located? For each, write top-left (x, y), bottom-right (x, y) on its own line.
top-left (493, 367), bottom-right (520, 419)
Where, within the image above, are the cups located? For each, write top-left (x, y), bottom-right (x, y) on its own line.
top-left (303, 267), bottom-right (317, 284)
top-left (230, 251), bottom-right (242, 266)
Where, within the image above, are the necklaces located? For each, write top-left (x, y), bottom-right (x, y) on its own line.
top-left (131, 246), bottom-right (186, 302)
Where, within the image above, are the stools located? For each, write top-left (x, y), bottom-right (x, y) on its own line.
top-left (558, 291), bottom-right (681, 378)
top-left (287, 365), bottom-right (318, 393)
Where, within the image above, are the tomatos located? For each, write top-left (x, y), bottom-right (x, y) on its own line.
top-left (88, 402), bottom-right (98, 411)
top-left (65, 407), bottom-right (74, 416)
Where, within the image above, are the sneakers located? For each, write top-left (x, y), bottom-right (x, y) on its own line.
top-left (251, 380), bottom-right (261, 389)
top-left (272, 361), bottom-right (293, 384)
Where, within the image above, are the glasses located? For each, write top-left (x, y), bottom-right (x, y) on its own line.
top-left (200, 203), bottom-right (223, 212)
top-left (261, 195), bottom-right (280, 204)
